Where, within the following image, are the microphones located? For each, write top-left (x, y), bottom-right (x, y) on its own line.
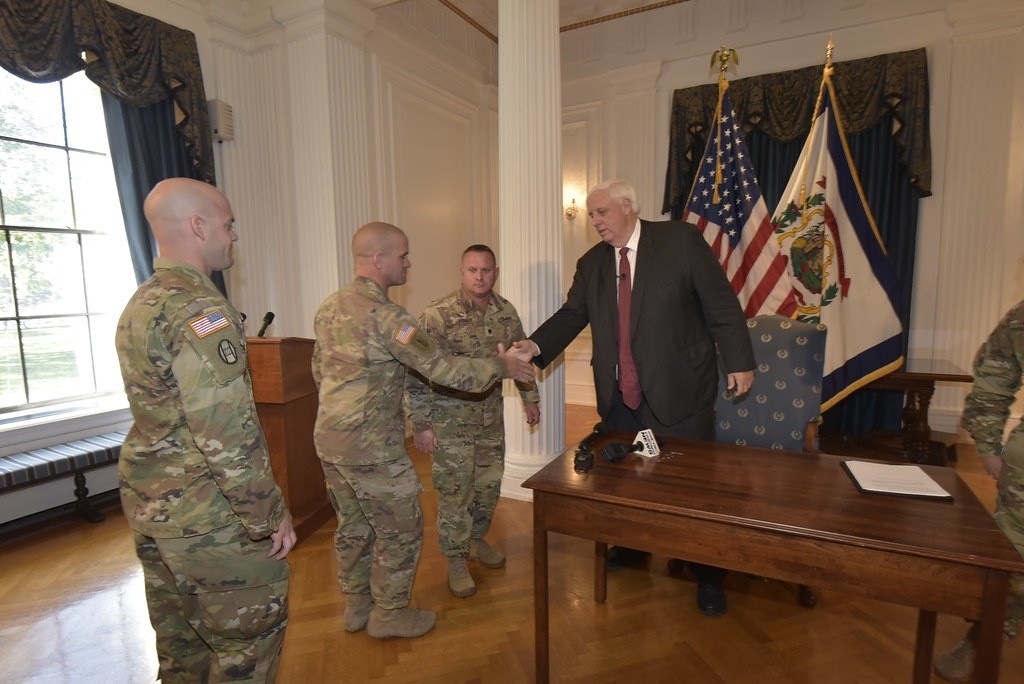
top-left (603, 429), bottom-right (664, 461)
top-left (257, 311), bottom-right (275, 337)
top-left (577, 418), bottom-right (612, 449)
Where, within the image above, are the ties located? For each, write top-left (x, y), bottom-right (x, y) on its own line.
top-left (617, 246), bottom-right (642, 410)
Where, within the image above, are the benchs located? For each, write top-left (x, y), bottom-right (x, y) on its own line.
top-left (0, 427), bottom-right (132, 524)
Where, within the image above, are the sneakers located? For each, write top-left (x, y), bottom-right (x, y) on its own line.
top-left (344, 594), bottom-right (372, 632)
top-left (366, 603), bottom-right (436, 638)
top-left (467, 539), bottom-right (506, 567)
top-left (933, 626), bottom-right (981, 682)
top-left (448, 555), bottom-right (475, 597)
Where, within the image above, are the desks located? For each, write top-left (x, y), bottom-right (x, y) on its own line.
top-left (863, 358), bottom-right (975, 465)
top-left (520, 429), bottom-right (1024, 684)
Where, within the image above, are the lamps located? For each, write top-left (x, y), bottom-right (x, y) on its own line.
top-left (565, 197), bottom-right (579, 220)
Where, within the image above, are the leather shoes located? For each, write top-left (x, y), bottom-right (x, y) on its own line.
top-left (697, 578), bottom-right (726, 615)
top-left (606, 546), bottom-right (651, 570)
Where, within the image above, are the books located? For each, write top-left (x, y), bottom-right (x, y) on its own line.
top-left (840, 460), bottom-right (955, 502)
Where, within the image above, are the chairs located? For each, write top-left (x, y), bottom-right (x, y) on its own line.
top-left (713, 313), bottom-right (827, 608)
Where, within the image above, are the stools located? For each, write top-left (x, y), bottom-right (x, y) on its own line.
top-left (929, 430), bottom-right (961, 467)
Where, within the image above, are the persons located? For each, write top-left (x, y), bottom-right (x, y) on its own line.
top-left (117, 177), bottom-right (298, 684)
top-left (933, 299), bottom-right (1024, 684)
top-left (311, 221), bottom-right (541, 640)
top-left (505, 177), bottom-right (757, 618)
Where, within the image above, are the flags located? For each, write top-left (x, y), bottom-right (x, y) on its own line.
top-left (682, 68), bottom-right (906, 414)
